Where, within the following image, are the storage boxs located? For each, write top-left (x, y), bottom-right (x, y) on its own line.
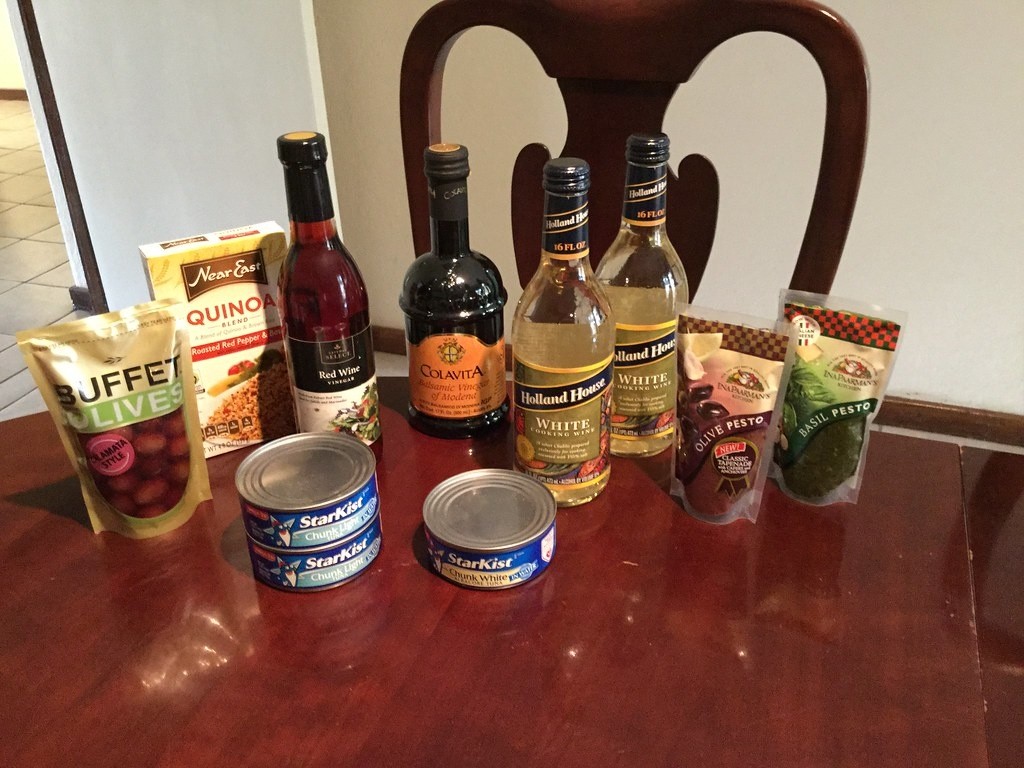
top-left (136, 220), bottom-right (295, 460)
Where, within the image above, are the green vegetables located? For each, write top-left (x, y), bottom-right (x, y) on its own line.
top-left (780, 366), bottom-right (833, 437)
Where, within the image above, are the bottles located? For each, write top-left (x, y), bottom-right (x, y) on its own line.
top-left (277, 130), bottom-right (384, 465)
top-left (596, 131), bottom-right (689, 458)
top-left (399, 142), bottom-right (510, 439)
top-left (510, 158), bottom-right (610, 508)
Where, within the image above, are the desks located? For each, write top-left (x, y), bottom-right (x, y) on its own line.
top-left (958, 447), bottom-right (1024, 768)
top-left (0, 376), bottom-right (988, 768)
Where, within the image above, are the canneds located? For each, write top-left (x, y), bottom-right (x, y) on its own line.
top-left (234, 431), bottom-right (382, 595)
top-left (423, 467), bottom-right (558, 592)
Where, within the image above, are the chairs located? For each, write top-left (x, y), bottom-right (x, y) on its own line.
top-left (399, 0), bottom-right (871, 305)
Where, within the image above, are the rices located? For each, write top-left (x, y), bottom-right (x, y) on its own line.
top-left (207, 362), bottom-right (293, 439)
